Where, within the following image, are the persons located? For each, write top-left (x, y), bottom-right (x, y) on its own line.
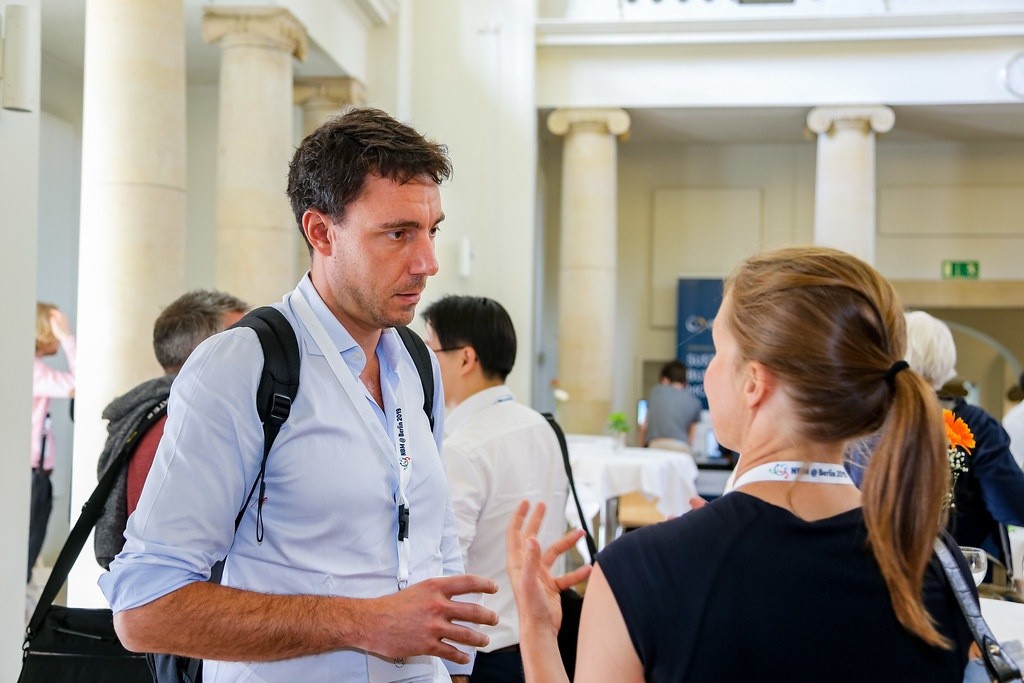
top-left (99, 106), bottom-right (500, 683)
top-left (94, 291), bottom-right (253, 683)
top-left (637, 360), bottom-right (702, 454)
top-left (421, 295), bottom-right (571, 683)
top-left (890, 309), bottom-right (1024, 603)
top-left (27, 303), bottom-right (75, 595)
top-left (506, 247), bottom-right (980, 683)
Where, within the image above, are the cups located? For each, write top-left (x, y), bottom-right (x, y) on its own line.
top-left (960, 547), bottom-right (988, 587)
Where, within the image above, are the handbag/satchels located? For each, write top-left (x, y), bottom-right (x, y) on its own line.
top-left (18, 605), bottom-right (158, 683)
top-left (557, 587), bottom-right (584, 683)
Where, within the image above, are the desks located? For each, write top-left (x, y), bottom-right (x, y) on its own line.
top-left (564, 434), bottom-right (700, 592)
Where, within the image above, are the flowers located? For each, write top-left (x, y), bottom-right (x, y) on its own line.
top-left (941, 409), bottom-right (975, 510)
